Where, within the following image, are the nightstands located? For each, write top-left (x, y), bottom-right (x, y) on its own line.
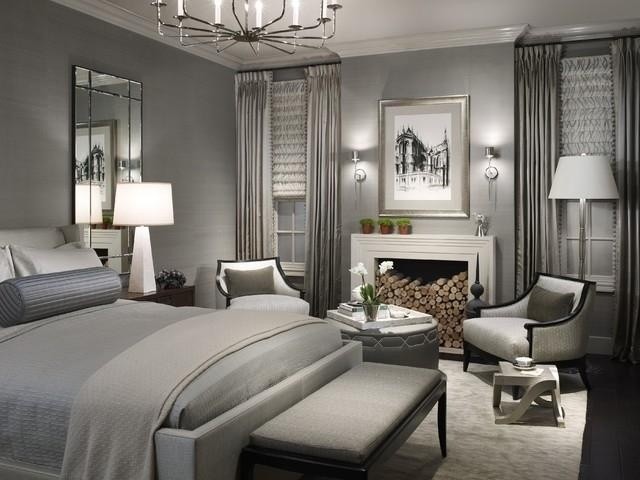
top-left (121, 284), bottom-right (196, 307)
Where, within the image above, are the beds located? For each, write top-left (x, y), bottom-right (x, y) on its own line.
top-left (0, 224), bottom-right (366, 480)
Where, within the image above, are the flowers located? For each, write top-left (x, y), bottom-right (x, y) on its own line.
top-left (347, 260), bottom-right (395, 304)
top-left (154, 265), bottom-right (188, 290)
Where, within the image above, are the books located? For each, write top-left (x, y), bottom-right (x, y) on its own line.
top-left (338, 301), bottom-right (389, 318)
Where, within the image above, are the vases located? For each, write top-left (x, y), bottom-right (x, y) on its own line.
top-left (362, 303), bottom-right (380, 321)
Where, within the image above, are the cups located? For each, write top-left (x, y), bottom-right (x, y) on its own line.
top-left (515, 356), bottom-right (533, 367)
top-left (102, 209), bottom-right (114, 226)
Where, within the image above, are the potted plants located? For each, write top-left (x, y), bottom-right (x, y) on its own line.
top-left (378, 218), bottom-right (396, 234)
top-left (396, 219), bottom-right (412, 234)
top-left (359, 217), bottom-right (373, 234)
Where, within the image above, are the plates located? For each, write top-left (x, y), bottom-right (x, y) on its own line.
top-left (513, 363), bottom-right (536, 370)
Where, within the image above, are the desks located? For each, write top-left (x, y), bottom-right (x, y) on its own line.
top-left (322, 316), bottom-right (439, 370)
top-left (493, 364), bottom-right (565, 429)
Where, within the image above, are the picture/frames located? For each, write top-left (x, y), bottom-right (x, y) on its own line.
top-left (375, 93), bottom-right (471, 219)
top-left (75, 117), bottom-right (118, 216)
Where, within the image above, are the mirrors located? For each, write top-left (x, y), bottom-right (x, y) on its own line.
top-left (70, 64), bottom-right (145, 286)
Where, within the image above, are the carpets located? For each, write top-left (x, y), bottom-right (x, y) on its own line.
top-left (300, 357), bottom-right (588, 479)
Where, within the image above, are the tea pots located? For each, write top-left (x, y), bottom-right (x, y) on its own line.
top-left (351, 284), bottom-right (384, 303)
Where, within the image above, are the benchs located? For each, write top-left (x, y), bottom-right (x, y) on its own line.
top-left (234, 360), bottom-right (448, 480)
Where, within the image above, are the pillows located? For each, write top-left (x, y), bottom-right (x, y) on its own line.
top-left (525, 285), bottom-right (575, 323)
top-left (0, 244), bottom-right (16, 283)
top-left (0, 264), bottom-right (123, 329)
top-left (9, 238), bottom-right (104, 278)
top-left (224, 264), bottom-right (277, 300)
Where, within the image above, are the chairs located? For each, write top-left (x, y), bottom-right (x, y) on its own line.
top-left (461, 270), bottom-right (597, 393)
top-left (213, 256), bottom-right (311, 316)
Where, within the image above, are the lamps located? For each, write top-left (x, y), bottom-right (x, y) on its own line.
top-left (480, 146), bottom-right (499, 200)
top-left (150, 0), bottom-right (343, 57)
top-left (351, 149), bottom-right (367, 184)
top-left (547, 151), bottom-right (623, 279)
top-left (112, 181), bottom-right (176, 294)
top-left (74, 181), bottom-right (104, 231)
